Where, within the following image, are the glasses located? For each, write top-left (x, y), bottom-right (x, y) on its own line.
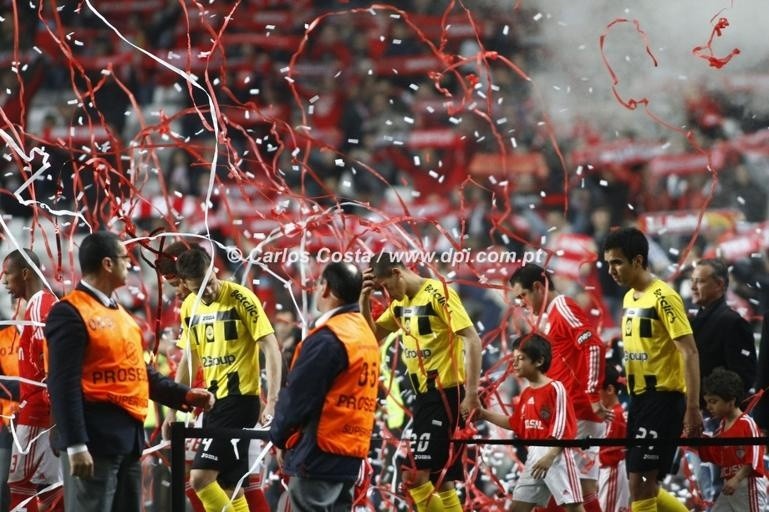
top-left (115, 255), bottom-right (130, 260)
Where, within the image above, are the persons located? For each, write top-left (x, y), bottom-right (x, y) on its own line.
top-left (0, 0), bottom-right (769, 511)
